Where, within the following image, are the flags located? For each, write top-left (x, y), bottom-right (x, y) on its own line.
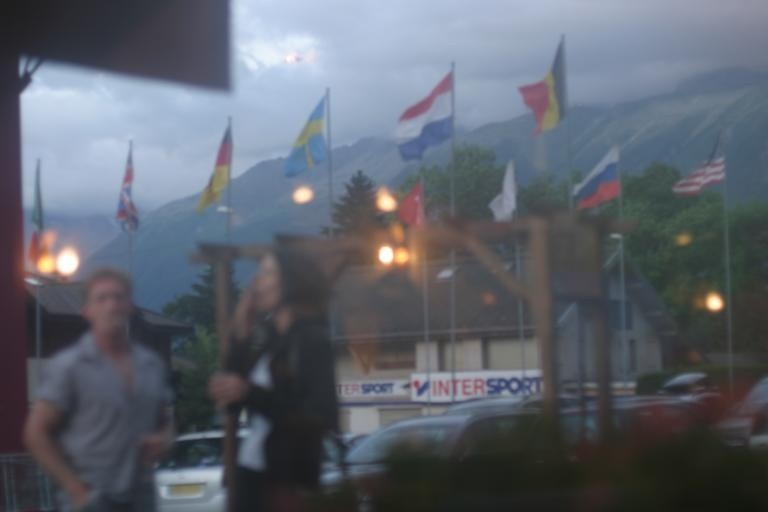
top-left (116, 139), bottom-right (138, 236)
top-left (396, 184), bottom-right (421, 229)
top-left (392, 70), bottom-right (454, 164)
top-left (27, 159), bottom-right (46, 273)
top-left (193, 118), bottom-right (232, 214)
top-left (281, 94), bottom-right (327, 181)
top-left (670, 134), bottom-right (725, 198)
top-left (572, 145), bottom-right (620, 216)
top-left (486, 159), bottom-right (516, 226)
top-left (518, 39), bottom-right (567, 138)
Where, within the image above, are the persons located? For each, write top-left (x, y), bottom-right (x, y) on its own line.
top-left (206, 239), bottom-right (339, 511)
top-left (22, 266), bottom-right (178, 510)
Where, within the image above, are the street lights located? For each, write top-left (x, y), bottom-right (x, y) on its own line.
top-left (21, 228), bottom-right (80, 385)
top-left (377, 191), bottom-right (432, 414)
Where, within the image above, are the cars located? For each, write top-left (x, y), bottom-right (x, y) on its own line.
top-left (313, 372), bottom-right (765, 512)
top-left (149, 427), bottom-right (228, 512)
